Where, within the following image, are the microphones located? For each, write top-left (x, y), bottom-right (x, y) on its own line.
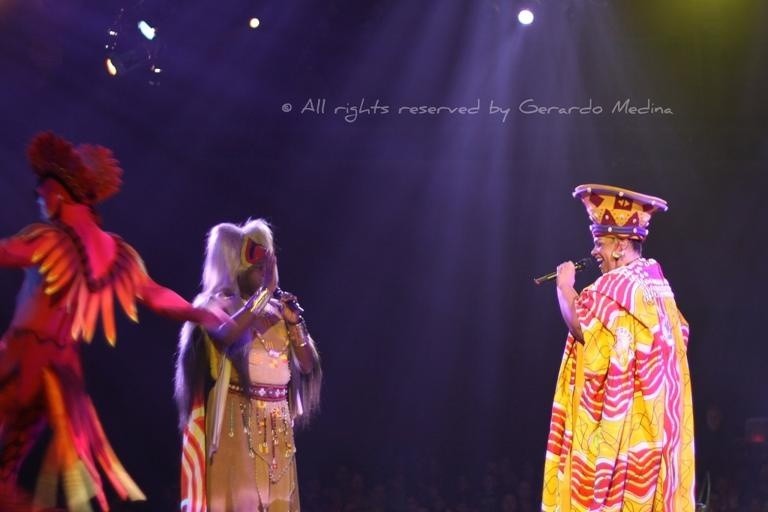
top-left (273, 287), bottom-right (305, 315)
top-left (531, 258), bottom-right (592, 289)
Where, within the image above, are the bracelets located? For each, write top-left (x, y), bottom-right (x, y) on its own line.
top-left (244, 286), bottom-right (273, 317)
top-left (285, 314), bottom-right (309, 348)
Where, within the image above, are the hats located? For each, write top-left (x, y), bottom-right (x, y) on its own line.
top-left (572, 184), bottom-right (668, 241)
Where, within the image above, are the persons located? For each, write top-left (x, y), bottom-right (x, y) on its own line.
top-left (540, 235), bottom-right (696, 511)
top-left (181, 217), bottom-right (317, 511)
top-left (0, 398), bottom-right (767, 510)
top-left (1, 170), bottom-right (234, 512)
